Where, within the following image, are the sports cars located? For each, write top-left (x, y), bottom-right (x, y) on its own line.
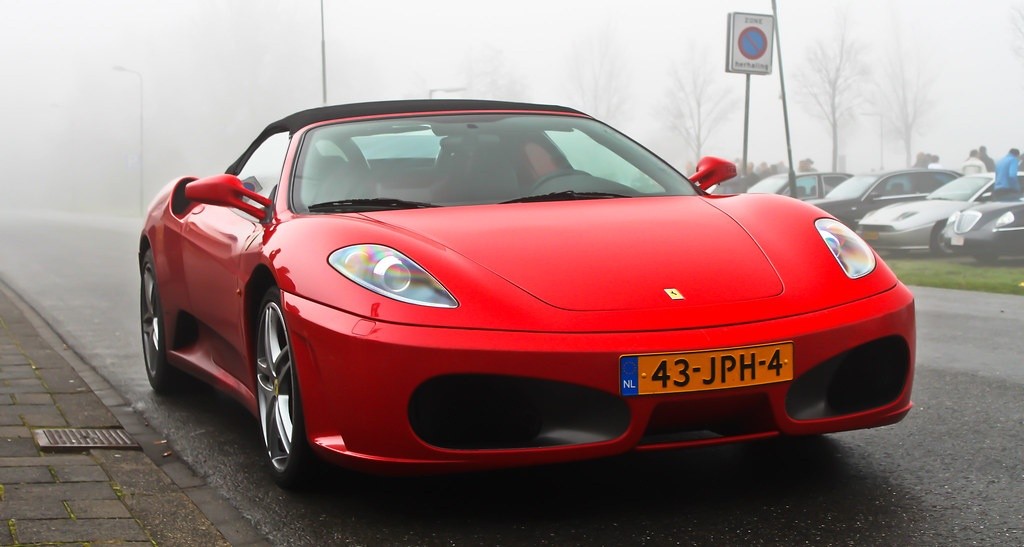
top-left (137, 100), bottom-right (918, 495)
top-left (856, 171), bottom-right (1024, 256)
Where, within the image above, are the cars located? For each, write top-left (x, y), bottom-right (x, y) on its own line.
top-left (748, 171), bottom-right (854, 205)
top-left (802, 167), bottom-right (965, 234)
top-left (938, 192), bottom-right (1024, 264)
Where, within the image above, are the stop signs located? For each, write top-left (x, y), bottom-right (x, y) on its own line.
top-left (726, 12), bottom-right (776, 75)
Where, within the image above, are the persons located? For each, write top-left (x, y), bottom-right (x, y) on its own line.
top-left (722, 141), bottom-right (1024, 200)
